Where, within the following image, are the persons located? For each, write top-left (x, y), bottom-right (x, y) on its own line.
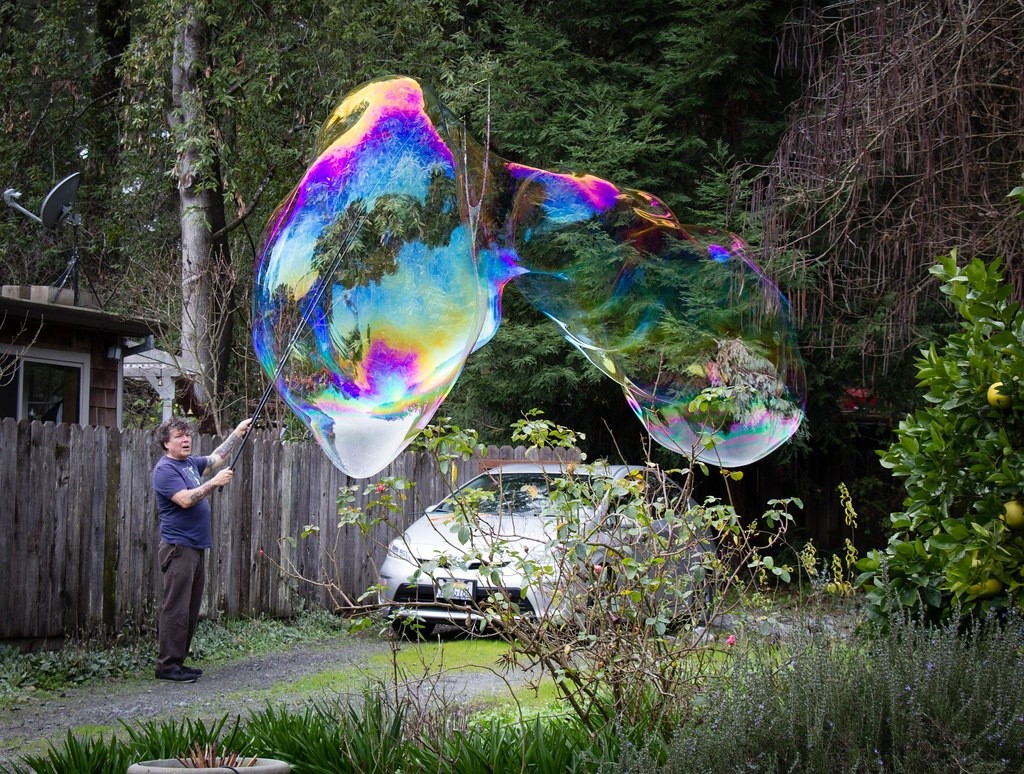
top-left (152, 417), bottom-right (253, 682)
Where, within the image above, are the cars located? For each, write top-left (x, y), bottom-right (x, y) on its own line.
top-left (375, 460), bottom-right (719, 639)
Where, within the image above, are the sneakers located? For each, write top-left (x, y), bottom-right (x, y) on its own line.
top-left (155, 668), bottom-right (197, 683)
top-left (181, 665), bottom-right (203, 676)
